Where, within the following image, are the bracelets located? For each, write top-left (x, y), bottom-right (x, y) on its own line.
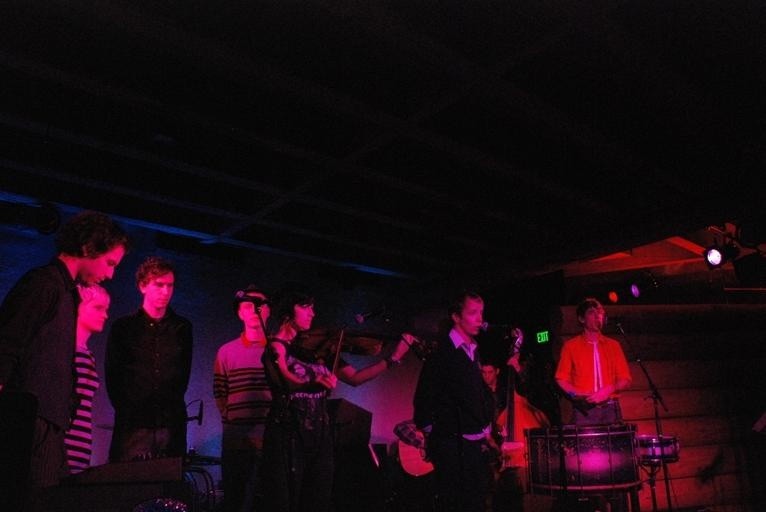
top-left (384, 350), bottom-right (398, 371)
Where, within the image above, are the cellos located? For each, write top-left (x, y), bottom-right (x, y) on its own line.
top-left (482, 328), bottom-right (555, 512)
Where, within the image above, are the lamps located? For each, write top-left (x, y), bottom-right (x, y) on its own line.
top-left (702, 233), bottom-right (741, 268)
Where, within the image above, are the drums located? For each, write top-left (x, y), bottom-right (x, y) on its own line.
top-left (524, 423), bottom-right (643, 494)
top-left (635, 433), bottom-right (680, 465)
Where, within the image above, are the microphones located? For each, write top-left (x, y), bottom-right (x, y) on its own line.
top-left (355, 310), bottom-right (375, 325)
top-left (235, 290), bottom-right (261, 304)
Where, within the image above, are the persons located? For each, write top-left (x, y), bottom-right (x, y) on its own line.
top-left (211, 283), bottom-right (277, 512)
top-left (553, 296), bottom-right (633, 426)
top-left (63, 281), bottom-right (111, 475)
top-left (409, 285), bottom-right (527, 512)
top-left (245, 290), bottom-right (417, 512)
top-left (477, 353), bottom-right (512, 443)
top-left (102, 253), bottom-right (195, 466)
top-left (0, 206), bottom-right (132, 512)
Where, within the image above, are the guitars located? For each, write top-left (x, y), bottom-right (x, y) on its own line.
top-left (393, 430), bottom-right (434, 476)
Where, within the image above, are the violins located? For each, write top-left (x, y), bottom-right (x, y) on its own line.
top-left (297, 323), bottom-right (433, 357)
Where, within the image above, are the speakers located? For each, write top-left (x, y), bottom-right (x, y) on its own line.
top-left (0, 387), bottom-right (39, 512)
